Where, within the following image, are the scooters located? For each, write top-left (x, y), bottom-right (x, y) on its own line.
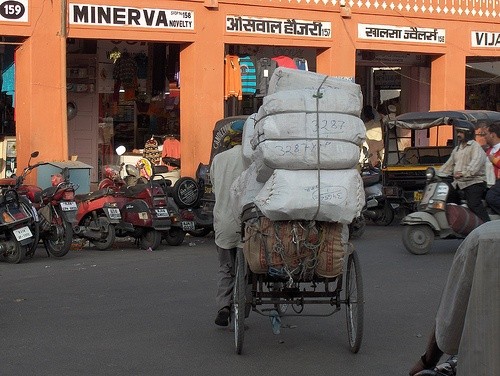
top-left (400, 166), bottom-right (500, 256)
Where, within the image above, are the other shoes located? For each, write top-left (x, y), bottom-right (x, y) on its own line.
top-left (215, 307), bottom-right (230, 327)
top-left (231, 320), bottom-right (248, 332)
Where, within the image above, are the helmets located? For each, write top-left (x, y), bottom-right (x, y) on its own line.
top-left (455, 121), bottom-right (475, 141)
top-left (231, 120), bottom-right (246, 132)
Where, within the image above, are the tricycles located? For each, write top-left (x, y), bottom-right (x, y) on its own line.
top-left (230, 246), bottom-right (365, 357)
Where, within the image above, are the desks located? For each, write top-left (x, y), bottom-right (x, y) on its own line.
top-left (120, 153), bottom-right (163, 179)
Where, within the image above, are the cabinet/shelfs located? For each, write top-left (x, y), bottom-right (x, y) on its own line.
top-left (113, 100), bottom-right (137, 153)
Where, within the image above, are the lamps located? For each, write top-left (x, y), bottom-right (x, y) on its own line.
top-left (106, 46), bottom-right (121, 63)
top-left (119, 86), bottom-right (125, 93)
top-left (165, 91), bottom-right (170, 95)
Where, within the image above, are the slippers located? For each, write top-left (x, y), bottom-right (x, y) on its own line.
top-left (269, 310), bottom-right (281, 334)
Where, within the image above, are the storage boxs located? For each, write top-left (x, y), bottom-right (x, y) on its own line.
top-left (137, 114), bottom-right (156, 129)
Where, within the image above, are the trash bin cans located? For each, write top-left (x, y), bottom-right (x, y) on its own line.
top-left (36, 160), bottom-right (94, 195)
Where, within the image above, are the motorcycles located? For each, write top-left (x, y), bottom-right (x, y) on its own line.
top-left (378, 110), bottom-right (500, 217)
top-left (348, 151), bottom-right (395, 237)
top-left (0, 144), bottom-right (205, 264)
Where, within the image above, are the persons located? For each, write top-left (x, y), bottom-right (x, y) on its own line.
top-left (484, 124), bottom-right (500, 215)
top-left (359, 105), bottom-right (385, 168)
top-left (408, 219), bottom-right (500, 376)
top-left (210, 119), bottom-right (251, 326)
top-left (437, 120), bottom-right (491, 223)
